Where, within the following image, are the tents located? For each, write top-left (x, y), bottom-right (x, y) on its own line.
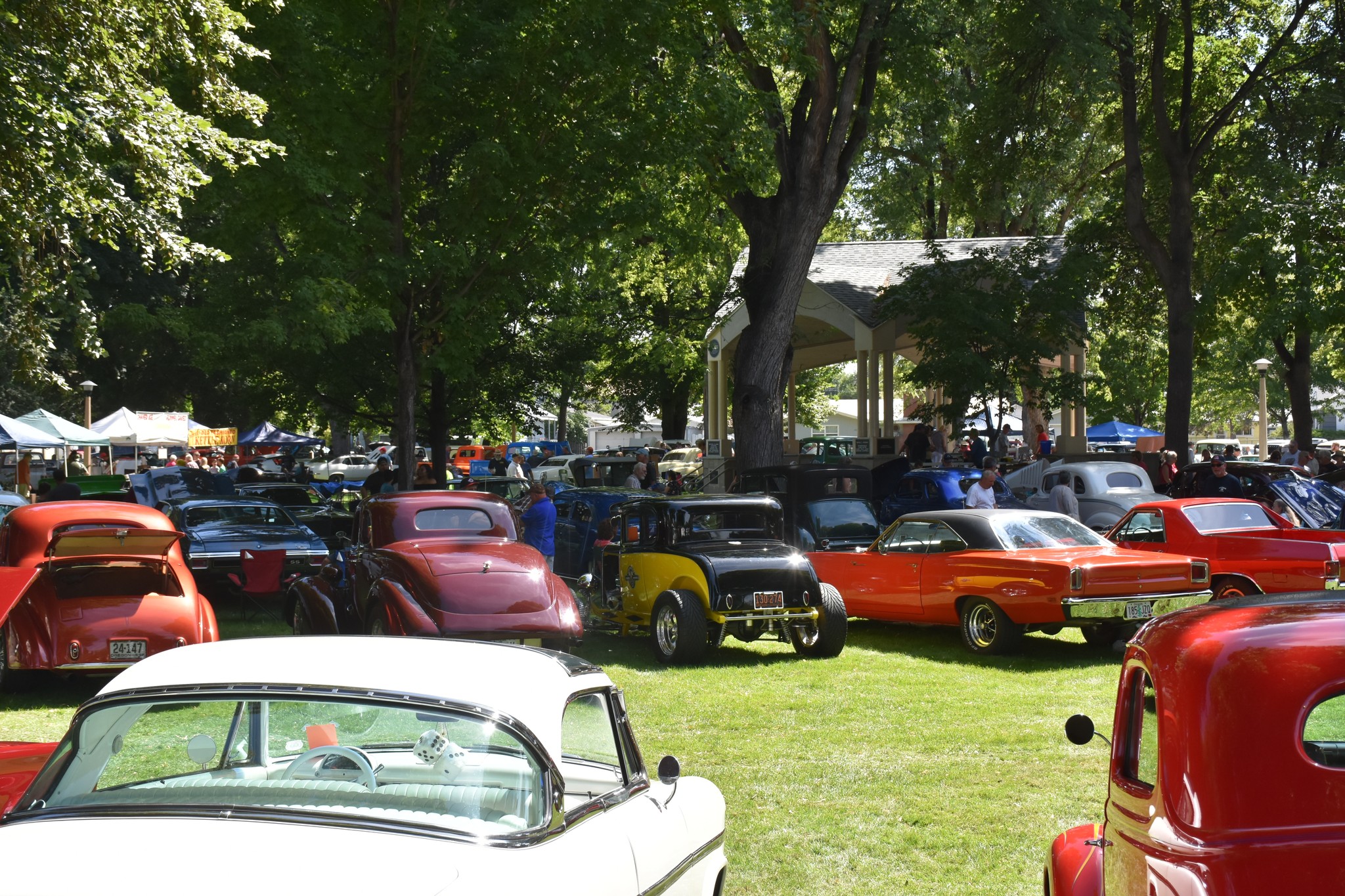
top-left (235, 421), bottom-right (326, 468)
top-left (0, 412), bottom-right (68, 493)
top-left (85, 406), bottom-right (187, 474)
top-left (171, 411), bottom-right (212, 450)
top-left (13, 408), bottom-right (113, 477)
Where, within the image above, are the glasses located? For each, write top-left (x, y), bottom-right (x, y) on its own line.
top-left (996, 467), bottom-right (997, 469)
top-left (643, 471), bottom-right (648, 473)
top-left (1210, 463), bottom-right (1224, 467)
top-left (1272, 457), bottom-right (1279, 460)
top-left (496, 454), bottom-right (501, 456)
top-left (1289, 446), bottom-right (1296, 448)
top-left (971, 428), bottom-right (976, 432)
top-left (615, 455), bottom-right (618, 457)
top-left (1172, 457), bottom-right (1177, 459)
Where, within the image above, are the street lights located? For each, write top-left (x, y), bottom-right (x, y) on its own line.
top-left (1254, 359), bottom-right (1273, 462)
top-left (79, 380), bottom-right (98, 476)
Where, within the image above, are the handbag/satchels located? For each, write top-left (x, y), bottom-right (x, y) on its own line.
top-left (1335, 480), bottom-right (1345, 491)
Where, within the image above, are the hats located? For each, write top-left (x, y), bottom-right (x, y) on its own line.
top-left (24, 452), bottom-right (34, 458)
top-left (377, 457), bottom-right (388, 464)
top-left (525, 484), bottom-right (556, 496)
top-left (378, 447), bottom-right (386, 452)
top-left (1298, 451), bottom-right (1312, 459)
top-left (1015, 438), bottom-right (1021, 442)
top-left (493, 448), bottom-right (503, 455)
top-left (917, 423), bottom-right (927, 429)
top-left (960, 441), bottom-right (968, 445)
top-left (461, 477), bottom-right (479, 488)
top-left (585, 447), bottom-right (594, 452)
top-left (1004, 423), bottom-right (1014, 431)
top-left (633, 447), bottom-right (650, 456)
top-left (1331, 450), bottom-right (1345, 462)
top-left (512, 452), bottom-right (521, 457)
top-left (532, 449), bottom-right (538, 455)
top-left (1234, 447), bottom-right (1242, 451)
top-left (941, 453), bottom-right (953, 462)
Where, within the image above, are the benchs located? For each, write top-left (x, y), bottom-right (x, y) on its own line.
top-left (58, 779), bottom-right (534, 833)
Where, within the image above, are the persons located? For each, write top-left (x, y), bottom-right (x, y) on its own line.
top-left (414, 422), bottom-right (1345, 653)
top-left (50, 470), bottom-right (80, 501)
top-left (166, 452), bottom-right (239, 474)
top-left (100, 452), bottom-right (116, 475)
top-left (50, 454), bottom-right (62, 467)
top-left (284, 461), bottom-right (305, 483)
top-left (62, 449), bottom-right (91, 476)
top-left (372, 448), bottom-right (393, 474)
top-left (36, 481), bottom-right (51, 502)
top-left (15, 452), bottom-right (33, 497)
top-left (354, 441), bottom-right (365, 455)
top-left (380, 469), bottom-right (394, 493)
top-left (256, 463), bottom-right (265, 472)
top-left (319, 445), bottom-right (325, 458)
top-left (327, 445), bottom-right (333, 454)
top-left (360, 457), bottom-right (399, 499)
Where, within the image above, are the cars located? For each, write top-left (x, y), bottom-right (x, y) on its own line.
top-left (0, 501), bottom-right (219, 695)
top-left (283, 490), bottom-right (582, 655)
top-left (1, 446), bottom-right (355, 604)
top-left (1042, 592), bottom-right (1345, 896)
top-left (731, 437), bottom-right (1345, 530)
top-left (0, 636), bottom-right (725, 896)
top-left (799, 509), bottom-right (1213, 655)
top-left (301, 441), bottom-right (706, 581)
top-left (575, 492), bottom-right (844, 664)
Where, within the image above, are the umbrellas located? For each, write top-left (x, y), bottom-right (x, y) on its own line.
top-left (1056, 420), bottom-right (1165, 444)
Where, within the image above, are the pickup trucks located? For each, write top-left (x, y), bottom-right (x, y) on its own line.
top-left (1021, 497), bottom-right (1345, 602)
